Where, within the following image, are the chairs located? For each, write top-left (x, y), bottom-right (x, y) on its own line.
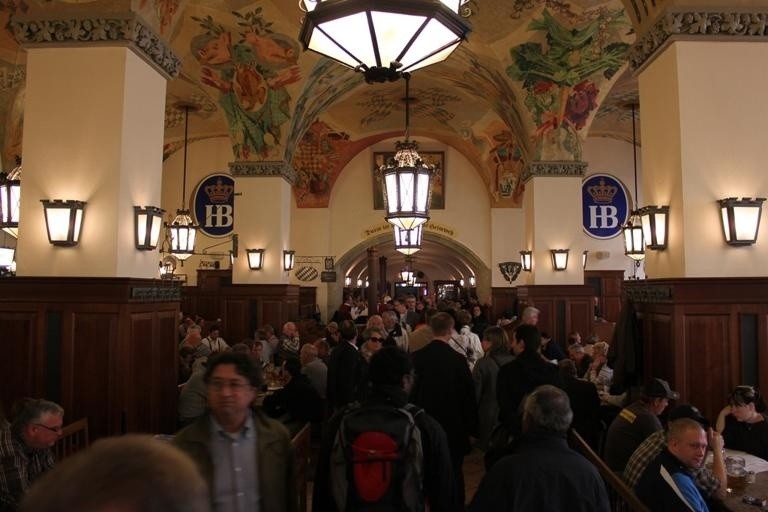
top-left (573, 429), bottom-right (642, 507)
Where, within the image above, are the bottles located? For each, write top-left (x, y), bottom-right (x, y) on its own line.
top-left (643, 378), bottom-right (680, 402)
top-left (671, 405), bottom-right (711, 431)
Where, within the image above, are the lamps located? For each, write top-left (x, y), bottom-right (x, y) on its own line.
top-left (519, 249), bottom-right (589, 274)
top-left (225, 247), bottom-right (296, 274)
top-left (1, 159), bottom-right (164, 284)
top-left (371, 67), bottom-right (440, 284)
top-left (630, 202), bottom-right (674, 254)
top-left (164, 102), bottom-right (205, 263)
top-left (294, 0), bottom-right (468, 84)
top-left (714, 193), bottom-right (767, 248)
top-left (616, 96), bottom-right (647, 263)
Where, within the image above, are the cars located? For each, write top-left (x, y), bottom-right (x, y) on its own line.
top-left (725, 453), bottom-right (746, 496)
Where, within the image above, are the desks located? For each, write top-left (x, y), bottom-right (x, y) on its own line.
top-left (701, 450), bottom-right (767, 506)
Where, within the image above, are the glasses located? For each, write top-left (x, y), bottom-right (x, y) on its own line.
top-left (294, 0), bottom-right (468, 84)
top-left (616, 96), bottom-right (647, 263)
top-left (371, 67), bottom-right (440, 284)
top-left (519, 249), bottom-right (589, 274)
top-left (714, 193), bottom-right (767, 248)
top-left (225, 247), bottom-right (296, 274)
top-left (630, 202), bottom-right (674, 254)
top-left (164, 102), bottom-right (205, 263)
top-left (1, 159), bottom-right (164, 284)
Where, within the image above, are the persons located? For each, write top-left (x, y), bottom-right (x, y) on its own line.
top-left (623, 403), bottom-right (727, 500)
top-left (174, 312), bottom-right (233, 433)
top-left (250, 295), bottom-right (633, 474)
top-left (600, 378), bottom-right (679, 477)
top-left (2, 396), bottom-right (64, 511)
top-left (29, 434), bottom-right (212, 510)
top-left (630, 418), bottom-right (708, 510)
top-left (714, 385), bottom-right (766, 459)
top-left (167, 350), bottom-right (308, 512)
top-left (469, 385), bottom-right (610, 511)
top-left (327, 346), bottom-right (459, 511)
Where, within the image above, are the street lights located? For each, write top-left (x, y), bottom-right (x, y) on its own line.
top-left (483, 422), bottom-right (510, 467)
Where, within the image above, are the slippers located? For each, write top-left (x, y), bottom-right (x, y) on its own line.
top-left (328, 402), bottom-right (428, 510)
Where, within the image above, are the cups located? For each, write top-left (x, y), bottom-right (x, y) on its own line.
top-left (207, 381), bottom-right (250, 392)
top-left (31, 422), bottom-right (66, 433)
top-left (368, 337), bottom-right (385, 344)
